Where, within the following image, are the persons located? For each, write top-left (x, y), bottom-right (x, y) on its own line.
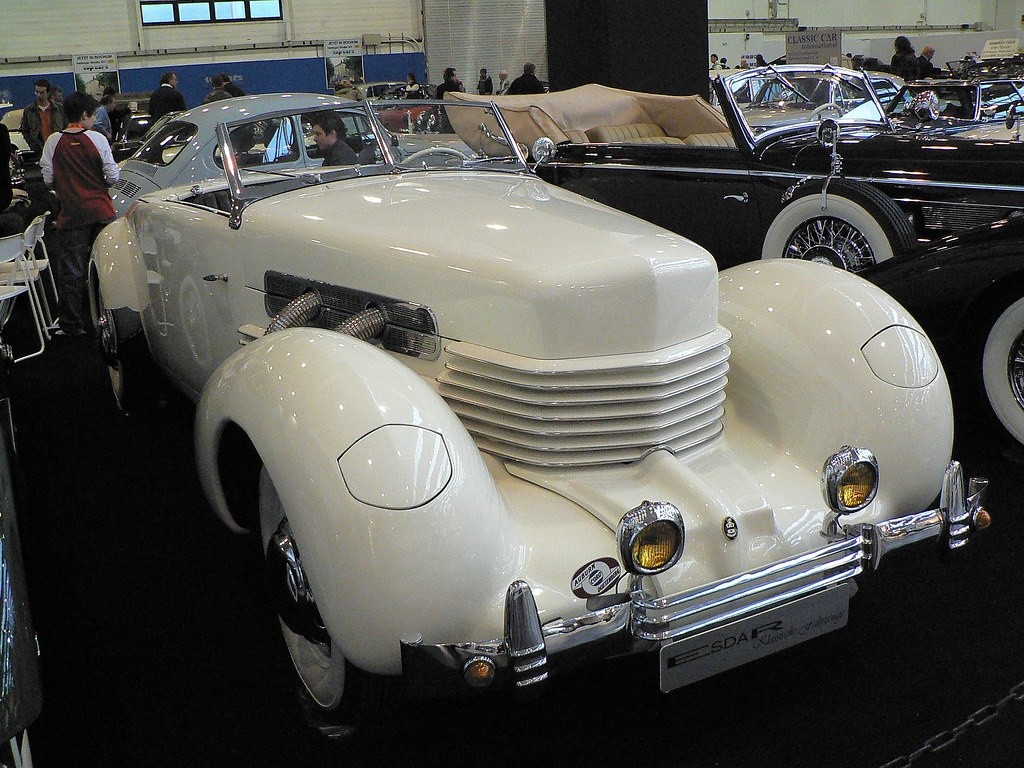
top-left (479, 63), bottom-right (545, 95)
top-left (842, 53), bottom-right (853, 70)
top-left (437, 63), bottom-right (466, 133)
top-left (311, 112), bottom-right (358, 166)
top-left (891, 36), bottom-right (940, 82)
top-left (711, 54), bottom-right (767, 69)
top-left (148, 71), bottom-right (187, 123)
top-left (352, 75), bottom-right (363, 84)
top-left (0, 78), bottom-right (131, 337)
top-left (406, 73), bottom-right (420, 99)
top-left (203, 72), bottom-right (245, 103)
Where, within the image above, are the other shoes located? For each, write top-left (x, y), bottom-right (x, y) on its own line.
top-left (65, 327), bottom-right (87, 336)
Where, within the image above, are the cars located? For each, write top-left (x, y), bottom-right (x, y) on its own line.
top-left (0, 79), bottom-right (442, 235)
top-left (443, 62), bottom-right (1024, 477)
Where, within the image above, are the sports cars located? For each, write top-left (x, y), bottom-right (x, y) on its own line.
top-left (86, 89), bottom-right (992, 735)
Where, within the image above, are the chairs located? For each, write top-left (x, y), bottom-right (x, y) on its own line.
top-left (0, 211), bottom-right (64, 363)
top-left (562, 123), bottom-right (741, 150)
top-left (229, 124), bottom-right (298, 166)
